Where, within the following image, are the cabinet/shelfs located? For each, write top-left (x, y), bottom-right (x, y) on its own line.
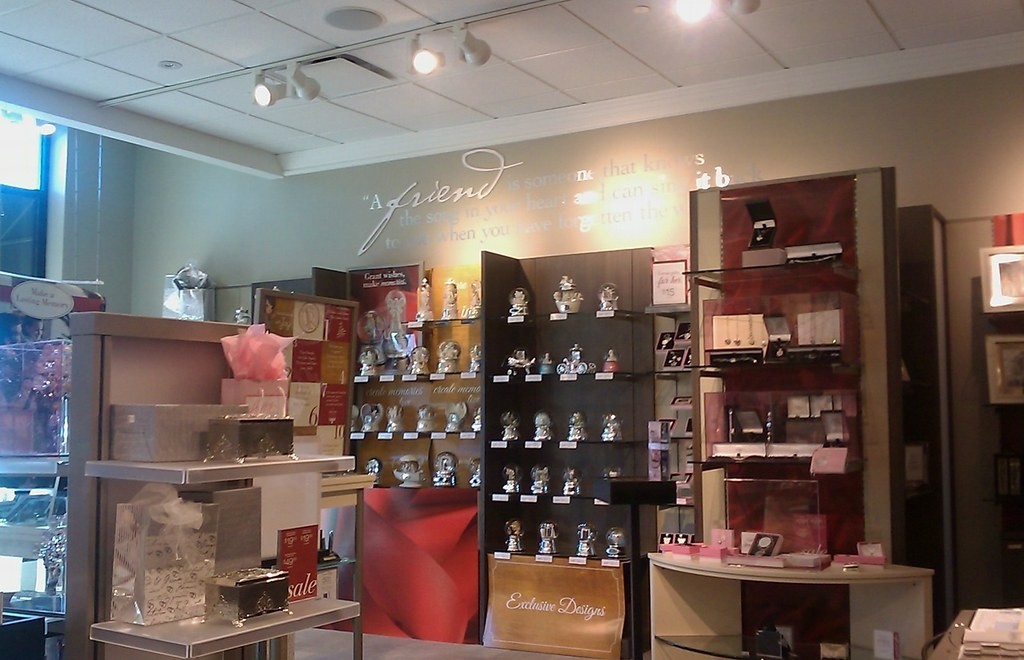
top-left (65, 169), bottom-right (1024, 660)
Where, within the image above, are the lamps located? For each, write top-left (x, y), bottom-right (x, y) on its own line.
top-left (453, 22), bottom-right (491, 66)
top-left (403, 35), bottom-right (445, 75)
top-left (254, 72), bottom-right (286, 106)
top-left (285, 62), bottom-right (320, 101)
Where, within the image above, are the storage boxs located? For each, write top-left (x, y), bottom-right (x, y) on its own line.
top-left (110, 406), bottom-right (248, 463)
top-left (209, 416), bottom-right (295, 464)
top-left (206, 568), bottom-right (288, 619)
